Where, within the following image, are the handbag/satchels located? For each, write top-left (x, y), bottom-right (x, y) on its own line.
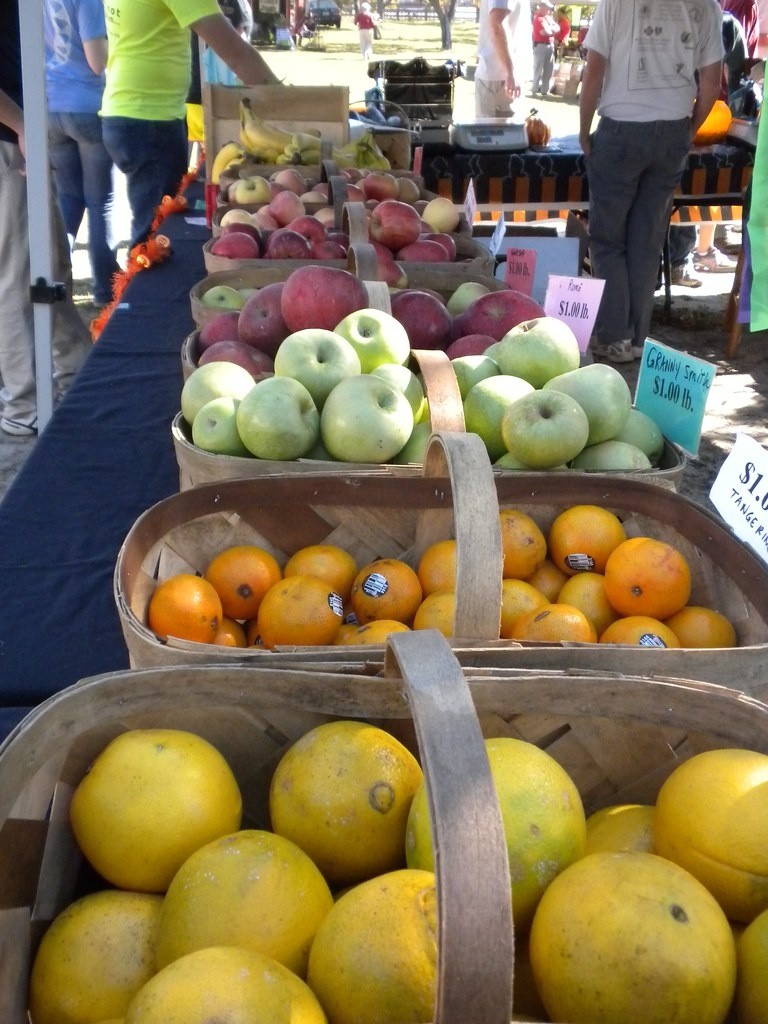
top-left (374, 25), bottom-right (381, 40)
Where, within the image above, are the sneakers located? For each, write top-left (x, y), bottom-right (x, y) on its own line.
top-left (590, 335), bottom-right (643, 362)
top-left (0, 387), bottom-right (67, 435)
top-left (691, 247), bottom-right (737, 273)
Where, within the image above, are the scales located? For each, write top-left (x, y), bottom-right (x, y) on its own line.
top-left (451, 120), bottom-right (529, 151)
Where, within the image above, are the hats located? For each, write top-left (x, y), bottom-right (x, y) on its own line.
top-left (541, 0), bottom-right (553, 9)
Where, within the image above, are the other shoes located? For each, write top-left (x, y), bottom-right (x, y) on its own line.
top-left (670, 263), bottom-right (702, 288)
top-left (92, 299), bottom-right (106, 307)
top-left (540, 92), bottom-right (552, 100)
top-left (532, 93), bottom-right (542, 100)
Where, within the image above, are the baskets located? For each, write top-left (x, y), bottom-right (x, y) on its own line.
top-left (180, 138), bottom-right (512, 385)
top-left (113, 432), bottom-right (768, 707)
top-left (171, 349), bottom-right (687, 493)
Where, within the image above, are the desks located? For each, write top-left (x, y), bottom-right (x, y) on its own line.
top-left (0, 126), bottom-right (757, 745)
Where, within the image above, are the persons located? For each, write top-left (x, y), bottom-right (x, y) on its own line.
top-left (0, 0), bottom-right (92, 435)
top-left (300, 17), bottom-right (317, 37)
top-left (473, 0), bottom-right (532, 118)
top-left (532, 0), bottom-right (560, 98)
top-left (101, 0), bottom-right (285, 256)
top-left (565, 0), bottom-right (725, 363)
top-left (554, 8), bottom-right (571, 49)
top-left (714, 0), bottom-right (760, 105)
top-left (218, 0), bottom-right (252, 42)
top-left (43, 0), bottom-right (123, 307)
top-left (354, 2), bottom-right (375, 60)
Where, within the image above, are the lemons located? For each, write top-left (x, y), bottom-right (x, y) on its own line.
top-left (30, 721), bottom-right (768, 1024)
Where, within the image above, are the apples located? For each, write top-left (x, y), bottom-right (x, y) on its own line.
top-left (180, 168), bottom-right (664, 473)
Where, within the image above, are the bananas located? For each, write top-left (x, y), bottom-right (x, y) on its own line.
top-left (523, 108), bottom-right (551, 146)
top-left (211, 96), bottom-right (392, 184)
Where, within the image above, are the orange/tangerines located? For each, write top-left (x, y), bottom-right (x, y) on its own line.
top-left (148, 506), bottom-right (736, 649)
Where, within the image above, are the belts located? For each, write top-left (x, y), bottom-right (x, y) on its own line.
top-left (537, 42), bottom-right (551, 44)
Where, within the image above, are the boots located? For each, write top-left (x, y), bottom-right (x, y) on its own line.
top-left (0, 627), bottom-right (768, 1024)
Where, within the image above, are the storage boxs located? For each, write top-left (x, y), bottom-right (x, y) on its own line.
top-left (354, 57), bottom-right (452, 126)
top-left (200, 79), bottom-right (350, 228)
